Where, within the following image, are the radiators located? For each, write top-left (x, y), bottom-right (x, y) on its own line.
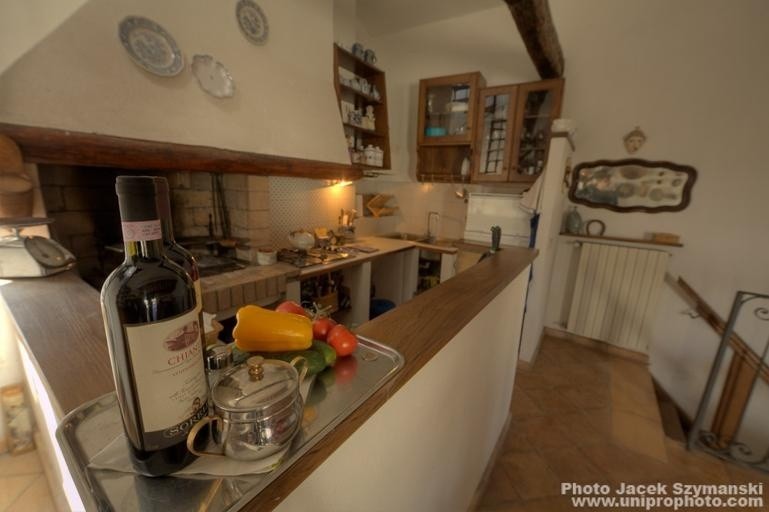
top-left (562, 241), bottom-right (669, 356)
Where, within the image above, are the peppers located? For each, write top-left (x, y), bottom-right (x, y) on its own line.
top-left (233, 305), bottom-right (313, 353)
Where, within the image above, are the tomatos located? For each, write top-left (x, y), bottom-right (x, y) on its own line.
top-left (275, 301), bottom-right (357, 357)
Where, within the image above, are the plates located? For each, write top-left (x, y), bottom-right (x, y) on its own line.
top-left (190, 55), bottom-right (240, 99)
top-left (234, 1), bottom-right (271, 47)
top-left (118, 13), bottom-right (187, 80)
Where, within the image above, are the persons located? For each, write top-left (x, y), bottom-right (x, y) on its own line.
top-left (578, 168), bottom-right (617, 206)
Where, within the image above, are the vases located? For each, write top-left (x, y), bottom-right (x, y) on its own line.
top-left (565, 206), bottom-right (582, 235)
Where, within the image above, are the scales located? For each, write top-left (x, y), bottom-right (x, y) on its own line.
top-left (0, 216), bottom-right (77, 278)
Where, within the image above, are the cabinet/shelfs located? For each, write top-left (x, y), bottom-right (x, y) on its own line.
top-left (415, 69), bottom-right (488, 184)
top-left (470, 76), bottom-right (566, 184)
top-left (334, 46), bottom-right (392, 171)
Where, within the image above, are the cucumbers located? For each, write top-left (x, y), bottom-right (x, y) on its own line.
top-left (232, 340), bottom-right (336, 373)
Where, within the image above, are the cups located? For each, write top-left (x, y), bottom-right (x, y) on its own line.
top-left (258, 250), bottom-right (278, 266)
top-left (366, 48), bottom-right (377, 65)
top-left (361, 117), bottom-right (369, 129)
top-left (351, 42), bottom-right (363, 58)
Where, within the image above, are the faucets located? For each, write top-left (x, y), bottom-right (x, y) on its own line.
top-left (426, 211), bottom-right (441, 237)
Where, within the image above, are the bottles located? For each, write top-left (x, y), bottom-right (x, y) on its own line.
top-left (426, 94), bottom-right (436, 112)
top-left (152, 176), bottom-right (203, 379)
top-left (350, 143), bottom-right (385, 166)
top-left (188, 352), bottom-right (310, 464)
top-left (366, 106), bottom-right (375, 131)
top-left (566, 204), bottom-right (581, 234)
top-left (100, 175), bottom-right (211, 477)
top-left (206, 343), bottom-right (234, 384)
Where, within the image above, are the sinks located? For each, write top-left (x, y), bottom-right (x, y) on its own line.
top-left (383, 231), bottom-right (420, 241)
top-left (418, 237), bottom-right (454, 248)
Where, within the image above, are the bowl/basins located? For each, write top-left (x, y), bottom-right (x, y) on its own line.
top-left (425, 127), bottom-right (447, 136)
